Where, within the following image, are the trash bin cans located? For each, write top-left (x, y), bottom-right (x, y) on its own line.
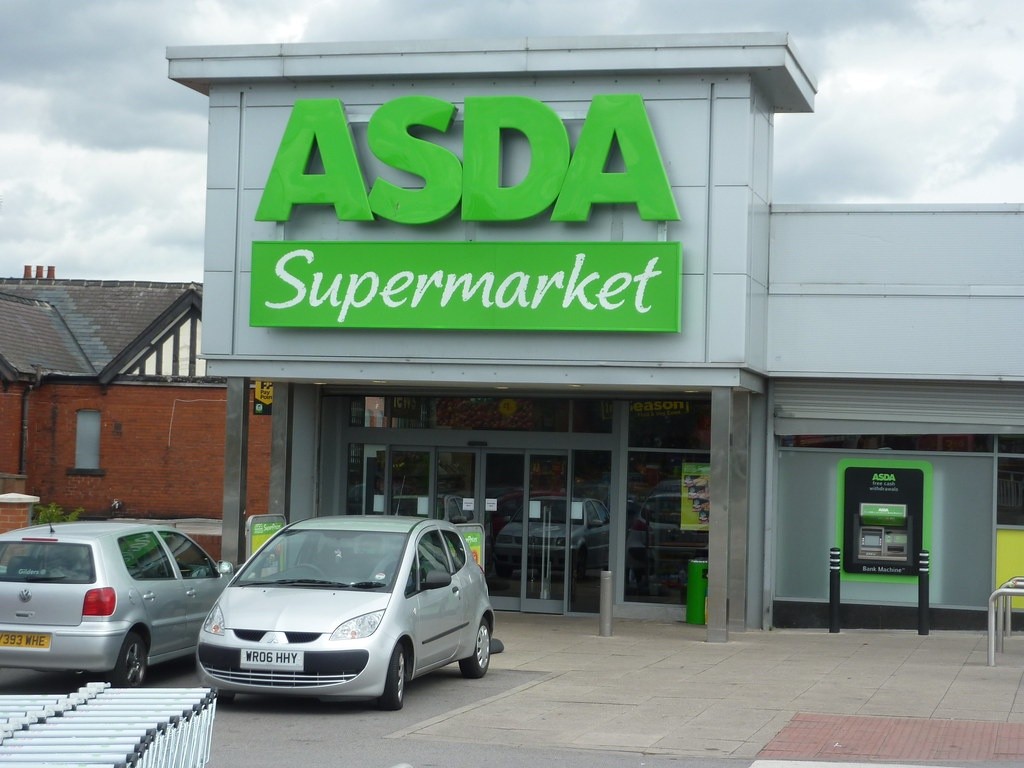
top-left (686, 558), bottom-right (708, 625)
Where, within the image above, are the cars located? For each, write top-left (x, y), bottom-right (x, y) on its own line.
top-left (0, 521), bottom-right (237, 687)
top-left (195, 513), bottom-right (494, 711)
top-left (347, 478), bottom-right (709, 599)
